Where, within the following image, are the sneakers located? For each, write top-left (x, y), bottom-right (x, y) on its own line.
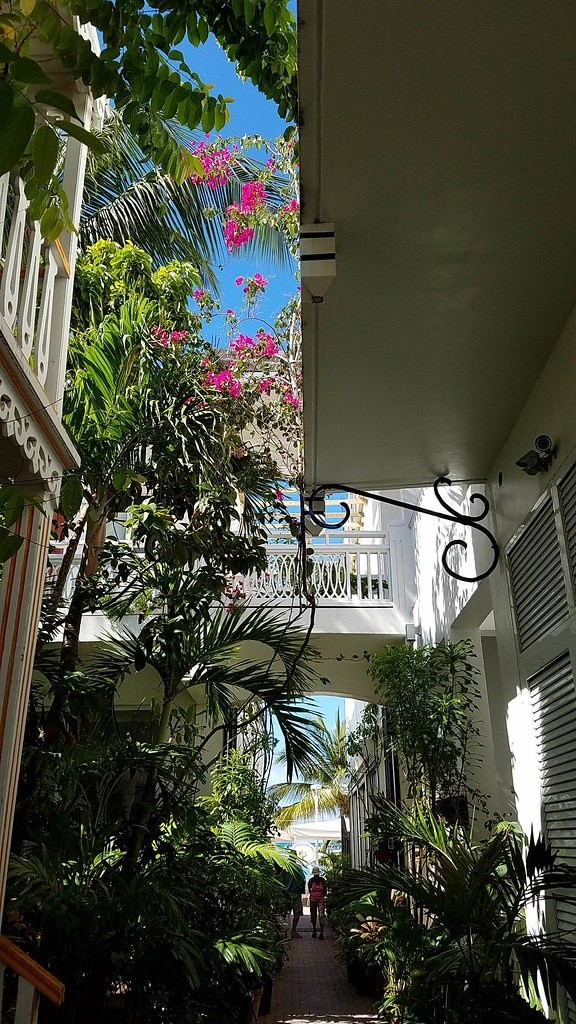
top-left (312, 932), bottom-right (317, 938)
top-left (291, 931), bottom-right (303, 939)
top-left (318, 934), bottom-right (324, 940)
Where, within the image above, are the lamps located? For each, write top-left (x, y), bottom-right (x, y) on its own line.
top-left (303, 492), bottom-right (327, 536)
top-left (296, 218), bottom-right (339, 303)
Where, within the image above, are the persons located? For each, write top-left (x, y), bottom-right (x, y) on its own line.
top-left (308, 867), bottom-right (327, 940)
top-left (283, 850), bottom-right (306, 939)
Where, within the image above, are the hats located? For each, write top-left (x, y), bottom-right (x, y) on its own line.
top-left (312, 867), bottom-right (321, 875)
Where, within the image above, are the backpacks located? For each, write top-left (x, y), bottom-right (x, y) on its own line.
top-left (311, 880), bottom-right (324, 902)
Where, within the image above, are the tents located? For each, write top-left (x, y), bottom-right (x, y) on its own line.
top-left (293, 817), bottom-right (351, 867)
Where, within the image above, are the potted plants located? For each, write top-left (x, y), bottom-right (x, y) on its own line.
top-left (319, 840), bottom-right (407, 998)
top-left (172, 812), bottom-right (302, 1022)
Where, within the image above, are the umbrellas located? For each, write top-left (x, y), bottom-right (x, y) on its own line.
top-left (265, 829), bottom-right (294, 842)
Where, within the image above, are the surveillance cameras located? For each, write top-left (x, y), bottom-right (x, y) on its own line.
top-left (535, 434), bottom-right (553, 452)
top-left (515, 450), bottom-right (541, 468)
